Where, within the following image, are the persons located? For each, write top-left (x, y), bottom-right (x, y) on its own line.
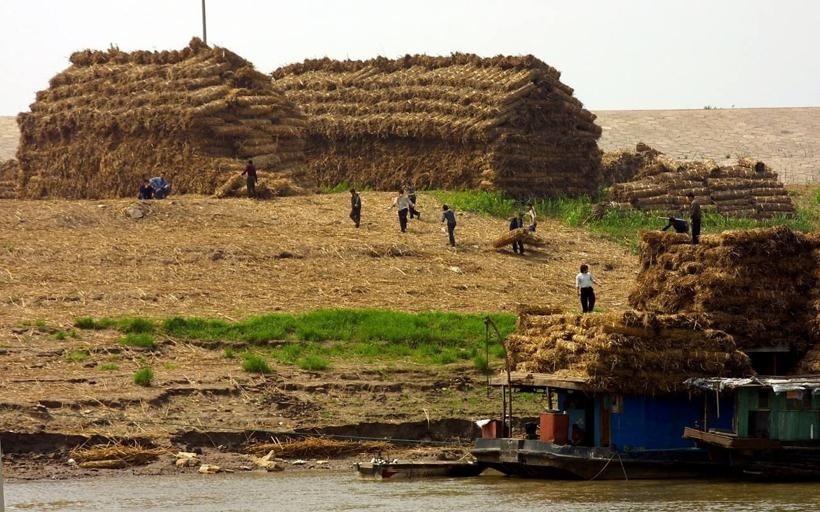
top-left (576, 264), bottom-right (602, 314)
top-left (406, 178), bottom-right (421, 220)
top-left (149, 172), bottom-right (171, 199)
top-left (685, 191), bottom-right (702, 244)
top-left (660, 217), bottom-right (689, 233)
top-left (138, 179), bottom-right (154, 200)
top-left (440, 203), bottom-right (457, 247)
top-left (348, 188), bottom-right (362, 228)
top-left (509, 211), bottom-right (526, 255)
top-left (240, 159), bottom-right (259, 198)
top-left (389, 187), bottom-right (415, 232)
top-left (526, 202), bottom-right (537, 232)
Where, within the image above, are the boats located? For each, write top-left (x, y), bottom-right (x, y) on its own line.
top-left (471, 227), bottom-right (820, 481)
top-left (681, 376), bottom-right (820, 481)
top-left (354, 450), bottom-right (489, 478)
